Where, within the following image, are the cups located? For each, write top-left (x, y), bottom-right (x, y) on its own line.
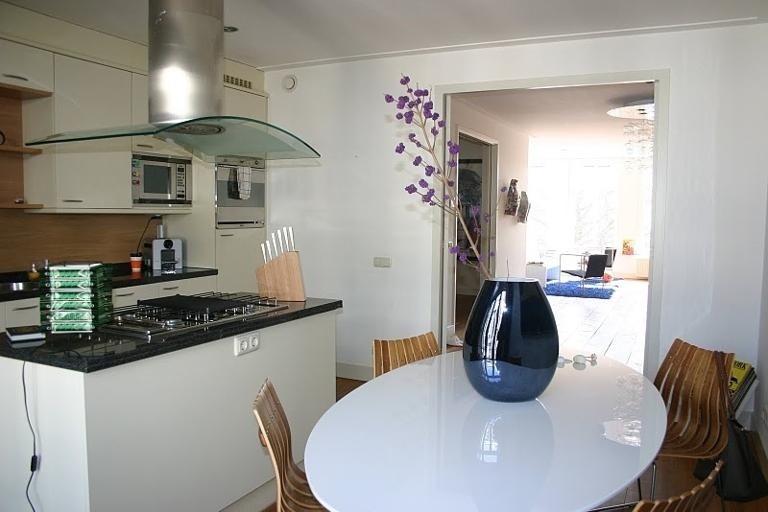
top-left (128, 251), bottom-right (143, 272)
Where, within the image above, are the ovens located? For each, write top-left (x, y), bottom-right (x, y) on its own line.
top-left (216, 155), bottom-right (269, 227)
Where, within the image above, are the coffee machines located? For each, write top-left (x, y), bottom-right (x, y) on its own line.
top-left (144, 224), bottom-right (183, 269)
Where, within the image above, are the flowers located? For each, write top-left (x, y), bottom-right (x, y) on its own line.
top-left (382, 70), bottom-right (492, 281)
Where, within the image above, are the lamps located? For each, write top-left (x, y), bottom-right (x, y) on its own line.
top-left (606, 99), bottom-right (655, 121)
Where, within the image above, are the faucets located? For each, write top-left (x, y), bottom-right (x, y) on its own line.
top-left (44, 259), bottom-right (49, 267)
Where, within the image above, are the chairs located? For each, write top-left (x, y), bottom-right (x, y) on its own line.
top-left (590, 338), bottom-right (735, 512)
top-left (374, 331), bottom-right (441, 377)
top-left (561, 254), bottom-right (608, 297)
top-left (632, 457), bottom-right (723, 512)
top-left (254, 376), bottom-right (328, 512)
top-left (604, 247), bottom-right (617, 268)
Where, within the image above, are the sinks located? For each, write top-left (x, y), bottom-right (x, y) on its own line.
top-left (0, 281), bottom-right (42, 293)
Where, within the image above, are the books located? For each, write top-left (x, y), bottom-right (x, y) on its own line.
top-left (6, 337), bottom-right (46, 349)
top-left (727, 358), bottom-right (758, 418)
top-left (5, 324), bottom-right (46, 343)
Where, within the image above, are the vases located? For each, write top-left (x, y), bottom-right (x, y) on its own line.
top-left (463, 278), bottom-right (559, 401)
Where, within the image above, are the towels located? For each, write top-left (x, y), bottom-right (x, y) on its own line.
top-left (237, 166), bottom-right (252, 201)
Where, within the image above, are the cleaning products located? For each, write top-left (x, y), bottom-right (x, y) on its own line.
top-left (27, 264), bottom-right (41, 282)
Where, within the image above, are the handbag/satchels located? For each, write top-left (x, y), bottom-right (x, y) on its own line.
top-left (692, 346), bottom-right (767, 503)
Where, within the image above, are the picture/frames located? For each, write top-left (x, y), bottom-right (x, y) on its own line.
top-left (458, 158), bottom-right (483, 262)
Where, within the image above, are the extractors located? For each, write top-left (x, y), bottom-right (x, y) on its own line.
top-left (24, 0), bottom-right (321, 163)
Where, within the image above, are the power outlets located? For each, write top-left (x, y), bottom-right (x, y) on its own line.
top-left (234, 331), bottom-right (260, 356)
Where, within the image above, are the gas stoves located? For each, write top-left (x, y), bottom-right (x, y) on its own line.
top-left (96, 290), bottom-right (288, 341)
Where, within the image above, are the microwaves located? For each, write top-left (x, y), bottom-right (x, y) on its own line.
top-left (131, 149), bottom-right (193, 204)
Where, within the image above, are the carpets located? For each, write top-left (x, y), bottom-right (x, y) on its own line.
top-left (543, 277), bottom-right (614, 299)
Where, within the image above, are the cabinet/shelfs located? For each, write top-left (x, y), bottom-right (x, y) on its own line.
top-left (24, 6), bottom-right (133, 214)
top-left (0, 0), bottom-right (53, 208)
